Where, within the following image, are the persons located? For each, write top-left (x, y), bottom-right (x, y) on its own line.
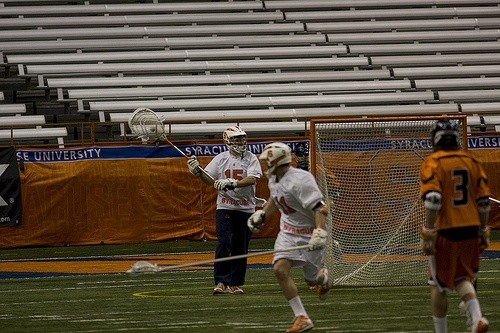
top-left (296, 144), bottom-right (342, 257)
top-left (419, 120), bottom-right (492, 333)
top-left (247, 142), bottom-right (330, 333)
top-left (187, 126), bottom-right (263, 295)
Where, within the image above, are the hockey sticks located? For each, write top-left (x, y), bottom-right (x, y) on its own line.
top-left (125, 244), bottom-right (313, 280)
top-left (127, 107), bottom-right (242, 205)
top-left (488, 196), bottom-right (500, 204)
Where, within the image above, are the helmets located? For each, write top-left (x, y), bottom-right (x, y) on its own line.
top-left (258, 142), bottom-right (292, 175)
top-left (223, 126), bottom-right (247, 155)
top-left (430, 119), bottom-right (460, 148)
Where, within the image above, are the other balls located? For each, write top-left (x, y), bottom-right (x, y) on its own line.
top-left (150, 126), bottom-right (156, 132)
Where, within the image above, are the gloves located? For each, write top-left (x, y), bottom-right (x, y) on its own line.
top-left (306, 228), bottom-right (328, 252)
top-left (420, 226), bottom-right (436, 255)
top-left (214, 177), bottom-right (237, 190)
top-left (187, 155), bottom-right (201, 176)
top-left (480, 227), bottom-right (490, 252)
top-left (247, 210), bottom-right (267, 233)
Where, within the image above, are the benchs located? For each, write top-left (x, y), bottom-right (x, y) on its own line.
top-left (0, 0), bottom-right (500, 149)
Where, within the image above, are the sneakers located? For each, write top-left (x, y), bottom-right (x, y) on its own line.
top-left (317, 268), bottom-right (331, 301)
top-left (228, 285), bottom-right (244, 294)
top-left (213, 282), bottom-right (226, 296)
top-left (285, 315), bottom-right (314, 333)
top-left (471, 319), bottom-right (490, 333)
